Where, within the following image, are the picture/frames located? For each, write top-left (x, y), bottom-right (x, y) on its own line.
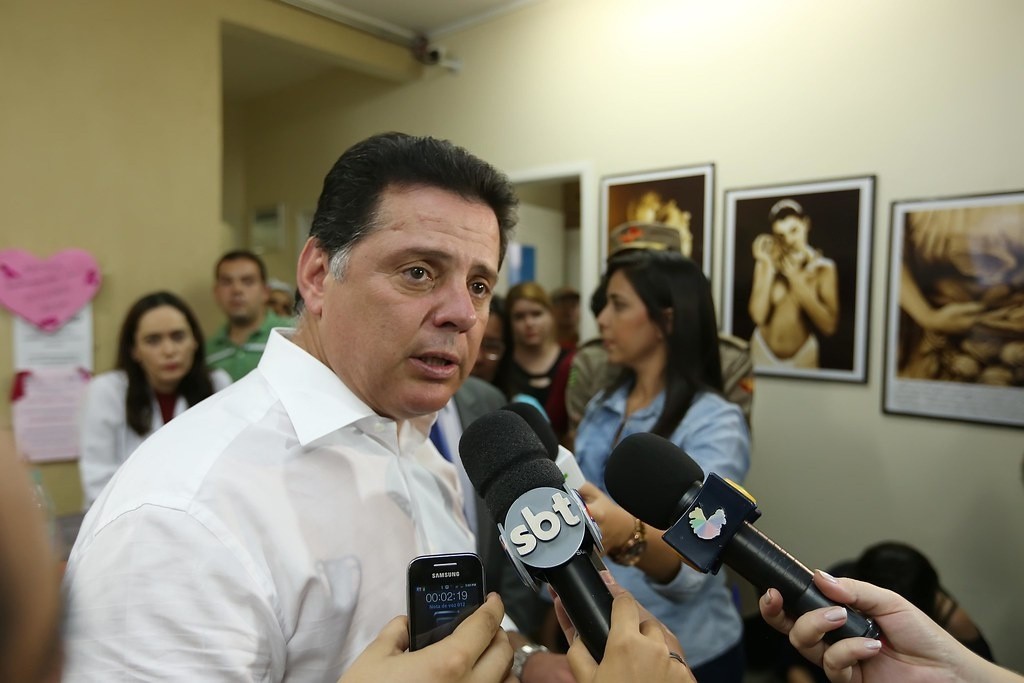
top-left (881, 189), bottom-right (1022, 430)
top-left (719, 174), bottom-right (874, 382)
top-left (596, 162), bottom-right (717, 287)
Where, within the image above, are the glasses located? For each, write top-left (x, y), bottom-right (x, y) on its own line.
top-left (478, 341), bottom-right (505, 361)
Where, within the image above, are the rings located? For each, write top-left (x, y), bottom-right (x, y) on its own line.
top-left (669, 650), bottom-right (687, 667)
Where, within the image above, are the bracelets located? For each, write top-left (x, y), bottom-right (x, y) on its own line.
top-left (510, 642), bottom-right (548, 681)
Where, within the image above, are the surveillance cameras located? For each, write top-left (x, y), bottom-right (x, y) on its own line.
top-left (423, 43), bottom-right (446, 65)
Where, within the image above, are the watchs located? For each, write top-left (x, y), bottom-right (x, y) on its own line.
top-left (608, 518), bottom-right (648, 568)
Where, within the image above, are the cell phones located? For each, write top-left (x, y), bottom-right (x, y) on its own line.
top-left (406, 553), bottom-right (485, 652)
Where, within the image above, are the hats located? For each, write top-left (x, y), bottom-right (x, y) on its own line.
top-left (268, 278), bottom-right (290, 292)
top-left (608, 224), bottom-right (682, 260)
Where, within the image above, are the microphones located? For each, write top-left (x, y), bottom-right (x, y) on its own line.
top-left (458, 393), bottom-right (617, 666)
top-left (604, 432), bottom-right (880, 644)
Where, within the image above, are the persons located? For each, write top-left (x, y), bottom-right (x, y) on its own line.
top-left (546, 572), bottom-right (698, 682)
top-left (78, 248), bottom-right (297, 517)
top-left (59, 129), bottom-right (578, 683)
top-left (471, 248), bottom-right (995, 683)
top-left (759, 567), bottom-right (1024, 683)
top-left (746, 199), bottom-right (840, 370)
